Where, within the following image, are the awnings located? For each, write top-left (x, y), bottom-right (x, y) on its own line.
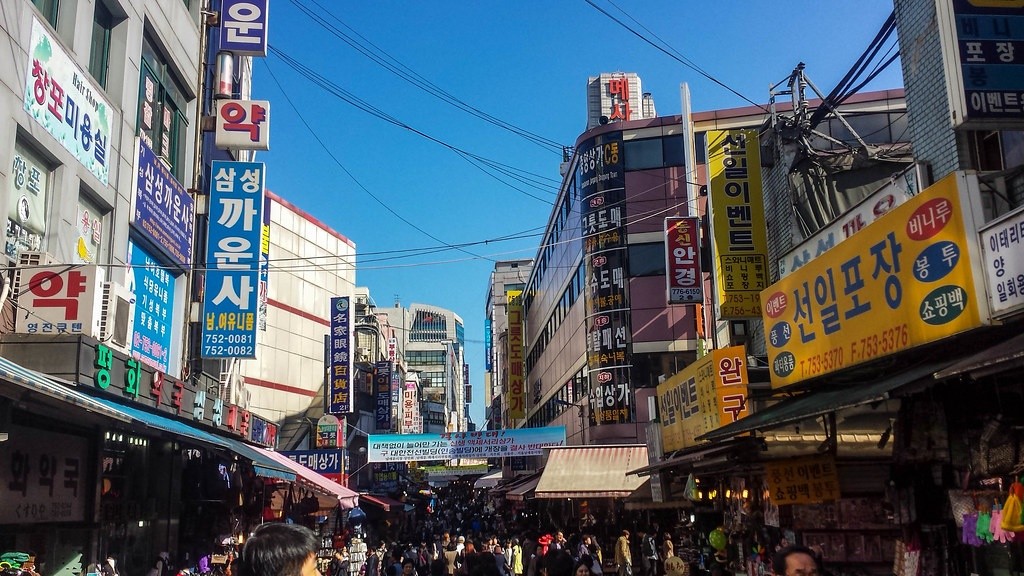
top-left (79, 391), bottom-right (234, 450)
top-left (933, 330), bottom-right (1024, 383)
top-left (242, 441), bottom-right (359, 509)
top-left (692, 346), bottom-right (987, 443)
top-left (535, 448), bottom-right (651, 498)
top-left (210, 434), bottom-right (298, 483)
top-left (504, 476), bottom-right (541, 502)
top-left (623, 442), bottom-right (737, 478)
top-left (361, 494), bottom-right (390, 511)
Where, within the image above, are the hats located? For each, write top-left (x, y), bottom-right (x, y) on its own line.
top-left (456, 536), bottom-right (465, 543)
top-left (539, 536), bottom-right (551, 545)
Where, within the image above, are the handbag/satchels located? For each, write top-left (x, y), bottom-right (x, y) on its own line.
top-left (331, 535), bottom-right (345, 549)
top-left (284, 483), bottom-right (319, 526)
top-left (948, 470), bottom-right (1010, 527)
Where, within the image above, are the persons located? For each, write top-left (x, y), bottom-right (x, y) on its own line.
top-left (580, 507), bottom-right (594, 529)
top-left (522, 529), bottom-right (604, 576)
top-left (330, 531), bottom-right (477, 576)
top-left (772, 544), bottom-right (818, 576)
top-left (661, 533), bottom-right (674, 561)
top-left (240, 521), bottom-right (322, 576)
top-left (636, 521), bottom-right (662, 576)
top-left (465, 534), bottom-right (523, 576)
top-left (615, 529), bottom-right (632, 576)
top-left (416, 478), bottom-right (518, 548)
